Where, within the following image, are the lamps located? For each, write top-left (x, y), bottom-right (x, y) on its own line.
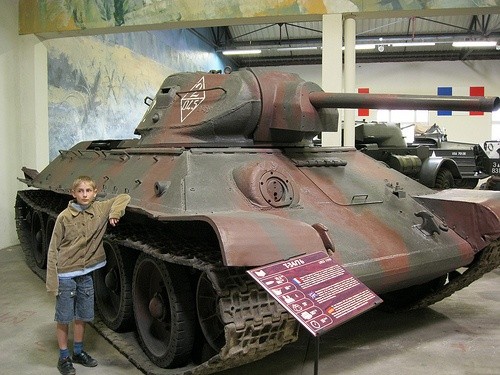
top-left (222, 48), bottom-right (261, 57)
top-left (391, 42), bottom-right (436, 47)
top-left (320, 43), bottom-right (376, 50)
top-left (451, 41), bottom-right (499, 47)
top-left (276, 46), bottom-right (316, 52)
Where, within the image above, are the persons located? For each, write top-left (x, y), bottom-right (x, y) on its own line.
top-left (46, 176), bottom-right (132, 375)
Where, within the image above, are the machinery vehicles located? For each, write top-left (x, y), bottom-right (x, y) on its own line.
top-left (13, 65), bottom-right (499, 375)
top-left (312, 118), bottom-right (499, 191)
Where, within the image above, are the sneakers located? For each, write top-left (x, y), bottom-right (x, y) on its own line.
top-left (57, 356), bottom-right (76, 375)
top-left (72, 350), bottom-right (98, 367)
